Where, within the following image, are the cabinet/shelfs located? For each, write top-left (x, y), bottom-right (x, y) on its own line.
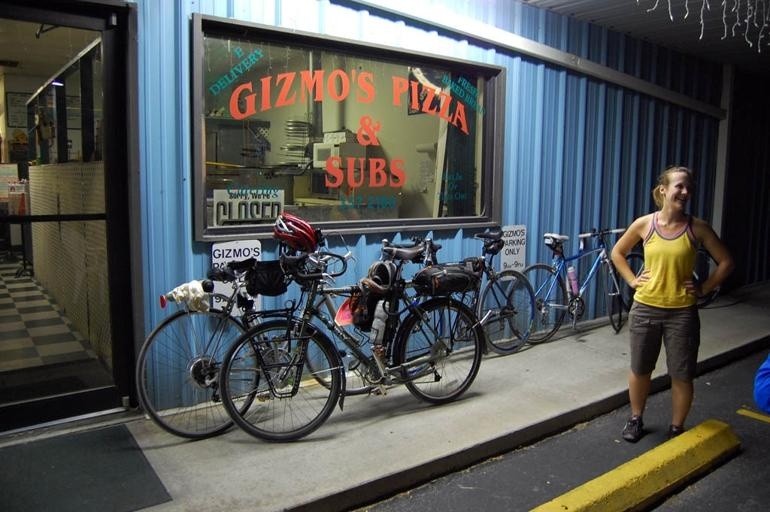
top-left (205, 116), bottom-right (271, 169)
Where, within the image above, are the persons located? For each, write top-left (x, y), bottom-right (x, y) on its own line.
top-left (752, 353), bottom-right (770, 415)
top-left (611, 164), bottom-right (736, 443)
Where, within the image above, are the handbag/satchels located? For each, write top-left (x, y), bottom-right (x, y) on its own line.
top-left (412, 262), bottom-right (482, 295)
top-left (245, 260), bottom-right (287, 297)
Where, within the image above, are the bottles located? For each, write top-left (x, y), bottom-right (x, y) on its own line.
top-left (240, 312), bottom-right (266, 348)
top-left (370, 299), bottom-right (390, 344)
top-left (566, 266), bottom-right (580, 296)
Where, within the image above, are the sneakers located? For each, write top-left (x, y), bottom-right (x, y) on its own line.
top-left (622, 415), bottom-right (645, 441)
top-left (668, 425), bottom-right (683, 437)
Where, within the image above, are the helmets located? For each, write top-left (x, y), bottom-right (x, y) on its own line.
top-left (273, 212), bottom-right (317, 253)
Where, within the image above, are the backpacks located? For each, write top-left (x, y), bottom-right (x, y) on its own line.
top-left (349, 260), bottom-right (401, 331)
